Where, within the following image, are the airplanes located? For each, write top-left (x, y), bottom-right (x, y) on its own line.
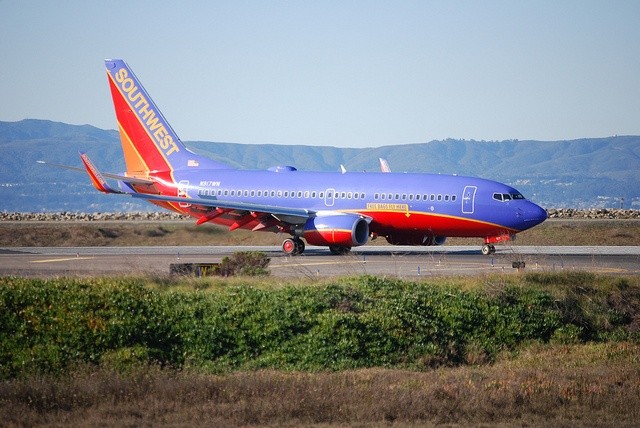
top-left (79, 59), bottom-right (547, 255)
